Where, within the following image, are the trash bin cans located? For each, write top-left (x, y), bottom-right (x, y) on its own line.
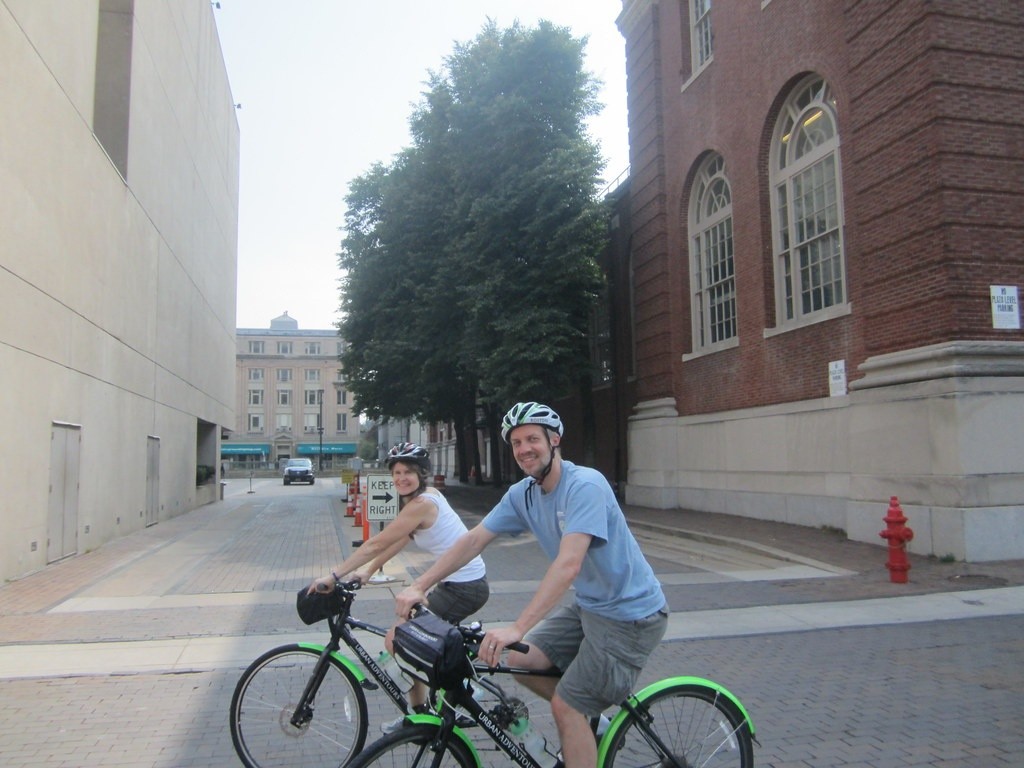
top-left (220, 483), bottom-right (227, 501)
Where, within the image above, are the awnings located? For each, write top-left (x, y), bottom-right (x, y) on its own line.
top-left (295, 443), bottom-right (357, 454)
top-left (220, 442), bottom-right (271, 454)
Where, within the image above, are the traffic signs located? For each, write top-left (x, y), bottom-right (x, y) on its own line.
top-left (365, 473), bottom-right (400, 523)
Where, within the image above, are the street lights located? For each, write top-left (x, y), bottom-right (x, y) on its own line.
top-left (317, 389), bottom-right (324, 471)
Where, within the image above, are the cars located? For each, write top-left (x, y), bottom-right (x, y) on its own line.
top-left (282, 457), bottom-right (316, 485)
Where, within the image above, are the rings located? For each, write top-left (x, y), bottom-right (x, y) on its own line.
top-left (489, 645), bottom-right (496, 650)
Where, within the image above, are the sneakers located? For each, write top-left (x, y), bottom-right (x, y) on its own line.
top-left (416, 711), bottom-right (439, 718)
top-left (379, 714), bottom-right (405, 734)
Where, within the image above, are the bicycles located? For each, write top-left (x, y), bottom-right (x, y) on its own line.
top-left (230, 581), bottom-right (601, 768)
top-left (340, 601), bottom-right (762, 768)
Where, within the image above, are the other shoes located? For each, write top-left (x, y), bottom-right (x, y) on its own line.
top-left (557, 714), bottom-right (626, 761)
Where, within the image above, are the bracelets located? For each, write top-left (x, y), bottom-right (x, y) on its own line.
top-left (331, 571), bottom-right (340, 583)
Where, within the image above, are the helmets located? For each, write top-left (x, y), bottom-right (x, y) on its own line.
top-left (501, 402), bottom-right (564, 444)
top-left (384, 442), bottom-right (430, 467)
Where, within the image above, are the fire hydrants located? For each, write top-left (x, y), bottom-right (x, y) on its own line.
top-left (878, 495), bottom-right (914, 585)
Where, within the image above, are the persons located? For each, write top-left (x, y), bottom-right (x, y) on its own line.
top-left (396, 402), bottom-right (670, 768)
top-left (306, 442), bottom-right (490, 734)
